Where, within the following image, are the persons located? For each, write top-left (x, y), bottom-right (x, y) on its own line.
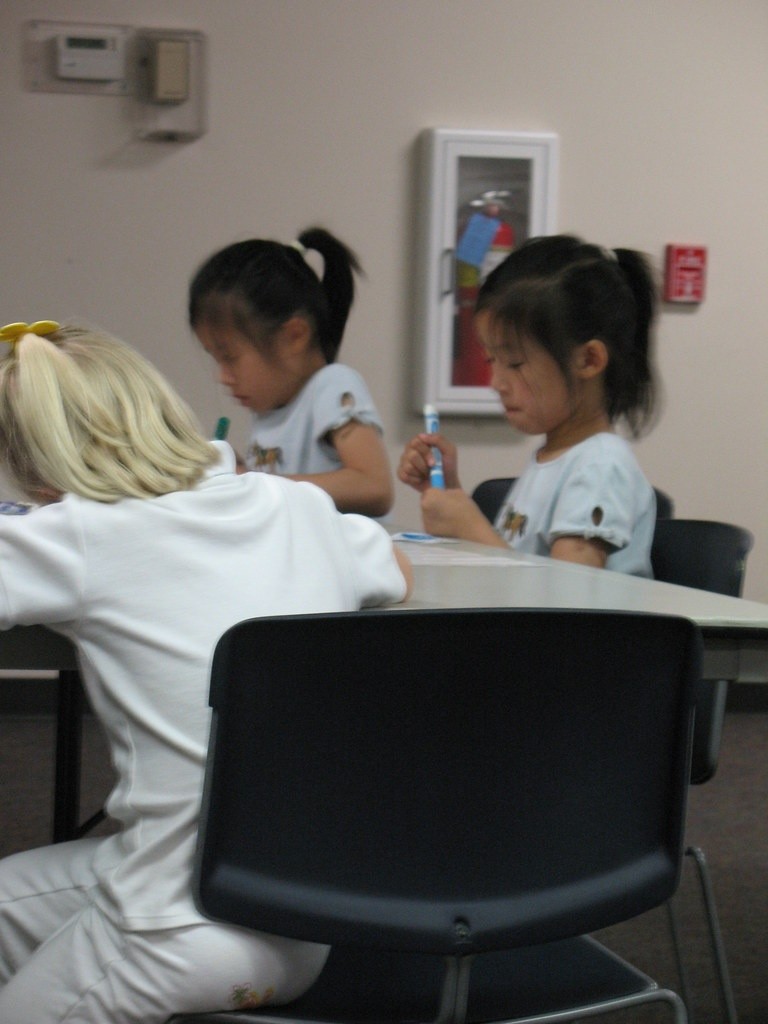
top-left (395, 233), bottom-right (659, 580)
top-left (0, 318), bottom-right (413, 1024)
top-left (187, 226), bottom-right (394, 519)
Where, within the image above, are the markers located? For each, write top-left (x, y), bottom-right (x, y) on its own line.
top-left (214, 416), bottom-right (230, 442)
top-left (423, 402), bottom-right (443, 491)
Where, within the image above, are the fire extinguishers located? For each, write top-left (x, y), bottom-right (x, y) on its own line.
top-left (455, 192), bottom-right (519, 389)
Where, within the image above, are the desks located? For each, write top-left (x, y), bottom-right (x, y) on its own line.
top-left (0, 531), bottom-right (768, 842)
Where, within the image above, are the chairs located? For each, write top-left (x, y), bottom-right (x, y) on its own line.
top-left (170, 608), bottom-right (706, 1024)
top-left (470, 477), bottom-right (672, 527)
top-left (650, 519), bottom-right (756, 1024)
